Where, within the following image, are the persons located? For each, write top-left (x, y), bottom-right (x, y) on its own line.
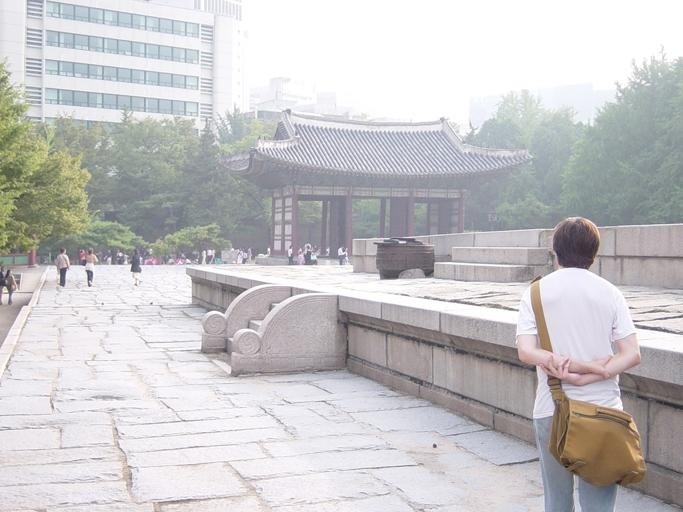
top-left (5, 269), bottom-right (18, 305)
top-left (127, 249), bottom-right (143, 286)
top-left (79, 249), bottom-right (86, 265)
top-left (344, 247), bottom-right (350, 264)
top-left (337, 246), bottom-right (346, 264)
top-left (511, 215), bottom-right (644, 512)
top-left (96, 246), bottom-right (257, 265)
top-left (0, 263), bottom-right (7, 305)
top-left (287, 241), bottom-right (321, 265)
top-left (85, 247), bottom-right (99, 287)
top-left (54, 246), bottom-right (71, 287)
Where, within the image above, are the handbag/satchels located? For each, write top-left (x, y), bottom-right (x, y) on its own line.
top-left (546, 397), bottom-right (647, 488)
